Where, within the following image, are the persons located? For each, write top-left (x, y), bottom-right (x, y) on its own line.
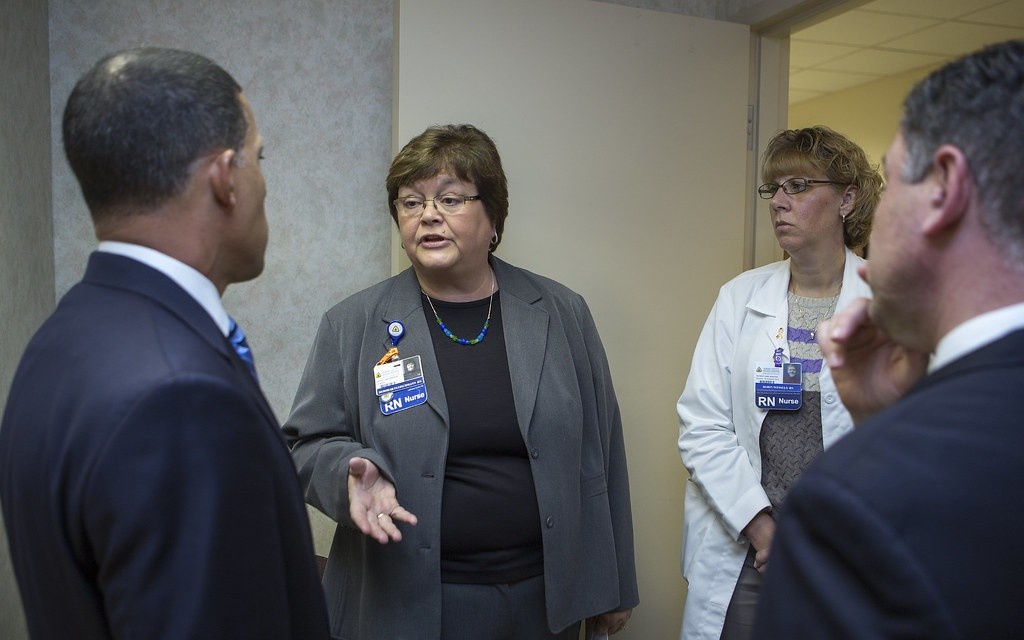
top-left (673, 126), bottom-right (927, 640)
top-left (739, 30), bottom-right (1021, 640)
top-left (0, 39), bottom-right (345, 640)
top-left (273, 122), bottom-right (643, 639)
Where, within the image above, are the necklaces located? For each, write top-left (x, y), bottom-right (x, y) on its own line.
top-left (415, 260), bottom-right (493, 345)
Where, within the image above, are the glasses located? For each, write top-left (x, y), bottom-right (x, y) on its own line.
top-left (758, 177), bottom-right (833, 199)
top-left (392, 194), bottom-right (483, 216)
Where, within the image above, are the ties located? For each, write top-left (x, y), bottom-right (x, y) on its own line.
top-left (227, 315), bottom-right (260, 388)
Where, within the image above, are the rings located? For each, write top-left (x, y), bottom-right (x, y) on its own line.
top-left (377, 513), bottom-right (384, 520)
